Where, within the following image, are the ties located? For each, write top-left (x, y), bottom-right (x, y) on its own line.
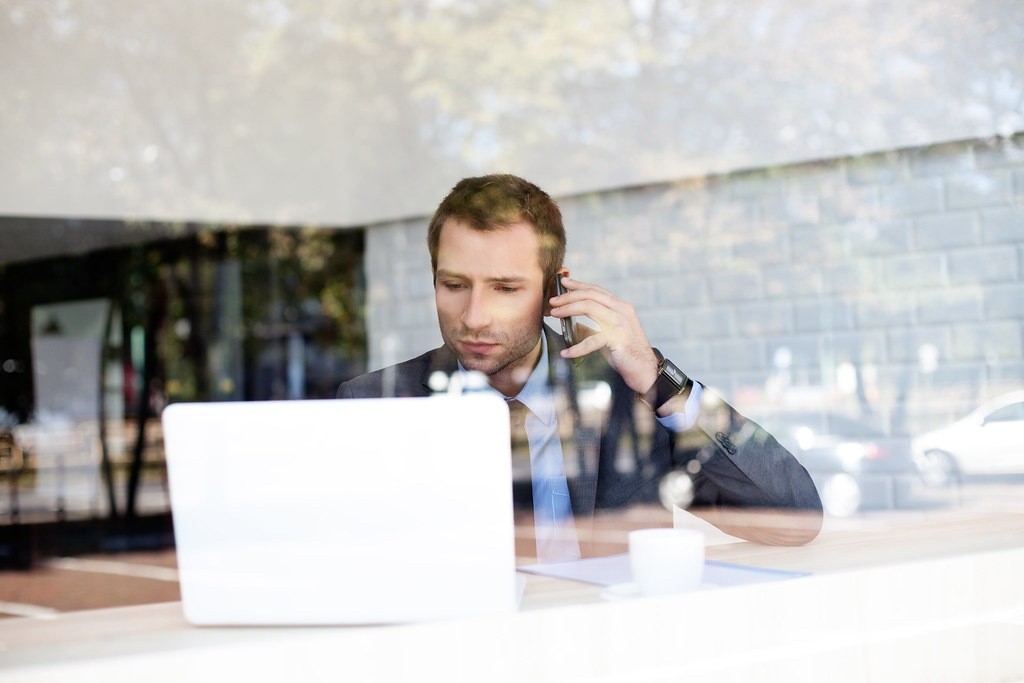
top-left (507, 397), bottom-right (539, 567)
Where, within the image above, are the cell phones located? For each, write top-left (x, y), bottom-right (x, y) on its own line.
top-left (555, 273), bottom-right (582, 369)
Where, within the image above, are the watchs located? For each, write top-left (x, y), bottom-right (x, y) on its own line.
top-left (635, 347), bottom-right (689, 415)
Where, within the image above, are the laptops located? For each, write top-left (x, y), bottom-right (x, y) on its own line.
top-left (159, 390), bottom-right (528, 629)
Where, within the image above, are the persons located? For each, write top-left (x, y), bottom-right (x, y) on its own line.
top-left (339, 175), bottom-right (824, 564)
